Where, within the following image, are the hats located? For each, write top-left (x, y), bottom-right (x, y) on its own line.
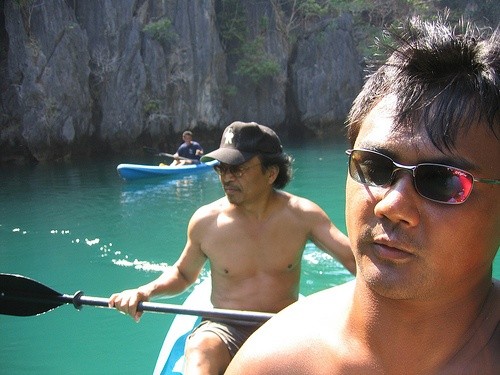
top-left (200, 120), bottom-right (284, 165)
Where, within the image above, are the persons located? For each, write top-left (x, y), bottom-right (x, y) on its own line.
top-left (224, 6), bottom-right (499, 375)
top-left (169, 130), bottom-right (203, 166)
top-left (108, 121), bottom-right (359, 375)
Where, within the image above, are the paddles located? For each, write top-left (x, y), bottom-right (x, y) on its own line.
top-left (0, 269), bottom-right (280, 327)
top-left (159, 152), bottom-right (201, 164)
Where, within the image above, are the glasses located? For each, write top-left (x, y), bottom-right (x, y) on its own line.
top-left (213, 164), bottom-right (268, 178)
top-left (345, 149), bottom-right (500, 205)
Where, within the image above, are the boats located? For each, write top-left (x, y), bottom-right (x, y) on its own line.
top-left (116, 158), bottom-right (221, 183)
